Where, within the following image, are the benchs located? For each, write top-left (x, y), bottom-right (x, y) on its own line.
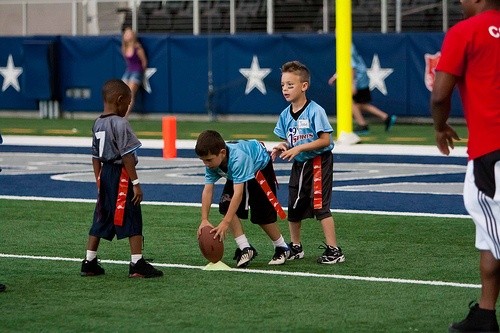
top-left (118, 0), bottom-right (465, 32)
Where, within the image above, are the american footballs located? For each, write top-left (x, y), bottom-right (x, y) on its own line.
top-left (198, 225), bottom-right (223, 264)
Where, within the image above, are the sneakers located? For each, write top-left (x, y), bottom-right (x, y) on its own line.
top-left (129, 258), bottom-right (163, 278)
top-left (268, 243), bottom-right (291, 265)
top-left (449, 301), bottom-right (500, 333)
top-left (317, 245), bottom-right (345, 264)
top-left (287, 243), bottom-right (304, 261)
top-left (81, 256), bottom-right (105, 276)
top-left (233, 244), bottom-right (257, 268)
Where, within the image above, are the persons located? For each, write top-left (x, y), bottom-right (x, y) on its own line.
top-left (431, 0), bottom-right (500, 333)
top-left (194, 129), bottom-right (293, 268)
top-left (270, 61), bottom-right (346, 264)
top-left (80, 79), bottom-right (163, 278)
top-left (121, 28), bottom-right (147, 118)
top-left (328, 41), bottom-right (396, 135)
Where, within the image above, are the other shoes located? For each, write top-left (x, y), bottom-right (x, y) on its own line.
top-left (385, 115), bottom-right (396, 132)
top-left (352, 125), bottom-right (371, 136)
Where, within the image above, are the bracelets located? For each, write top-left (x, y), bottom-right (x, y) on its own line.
top-left (132, 179), bottom-right (138, 186)
